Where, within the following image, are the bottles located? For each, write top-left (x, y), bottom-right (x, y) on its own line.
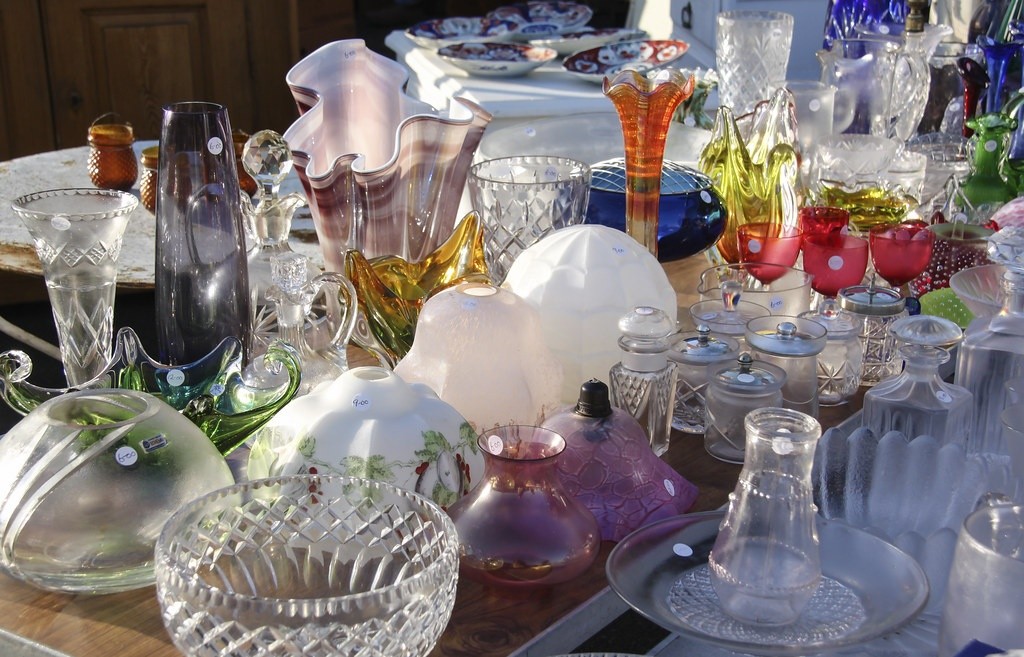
top-left (392, 264), bottom-right (1024, 657)
top-left (0, 384), bottom-right (241, 595)
top-left (240, 360), bottom-right (490, 565)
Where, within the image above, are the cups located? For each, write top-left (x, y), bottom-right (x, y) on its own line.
top-left (470, 151), bottom-right (593, 283)
top-left (669, 1), bottom-right (981, 461)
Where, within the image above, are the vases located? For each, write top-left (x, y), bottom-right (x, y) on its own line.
top-left (9, 0), bottom-right (1024, 390)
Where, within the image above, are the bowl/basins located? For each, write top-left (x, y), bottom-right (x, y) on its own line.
top-left (155, 473), bottom-right (460, 657)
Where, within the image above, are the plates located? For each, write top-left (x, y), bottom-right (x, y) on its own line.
top-left (404, 16), bottom-right (518, 50)
top-left (607, 502), bottom-right (932, 652)
top-left (527, 25), bottom-right (645, 57)
top-left (560, 39), bottom-right (690, 85)
top-left (486, 1), bottom-right (593, 35)
top-left (435, 41), bottom-right (557, 77)
